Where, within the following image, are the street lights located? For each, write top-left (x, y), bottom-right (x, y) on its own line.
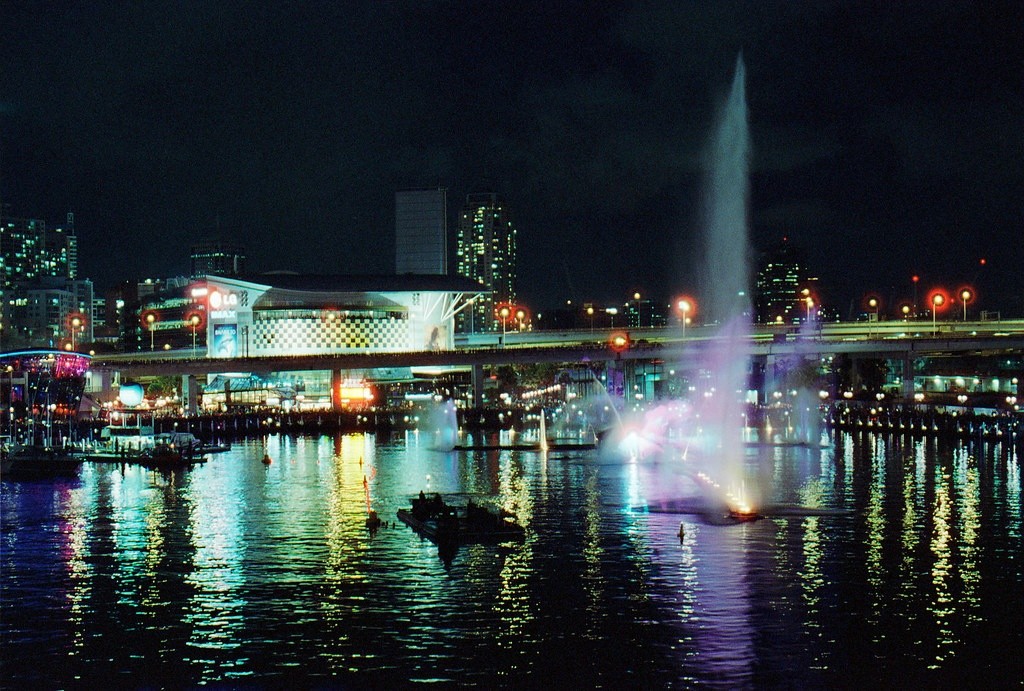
top-left (678, 301), bottom-right (688, 340)
top-left (587, 307), bottom-right (594, 335)
top-left (806, 297), bottom-right (813, 323)
top-left (148, 315), bottom-right (155, 351)
top-left (501, 308), bottom-right (509, 345)
top-left (634, 293), bottom-right (641, 328)
top-left (933, 296), bottom-right (943, 337)
top-left (517, 312), bottom-right (524, 334)
top-left (192, 316), bottom-right (200, 357)
top-left (71, 319), bottom-right (80, 352)
top-left (902, 306), bottom-right (911, 337)
top-left (870, 299), bottom-right (879, 340)
top-left (962, 290), bottom-right (971, 322)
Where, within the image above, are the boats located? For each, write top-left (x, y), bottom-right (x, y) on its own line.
top-left (395, 505), bottom-right (527, 548)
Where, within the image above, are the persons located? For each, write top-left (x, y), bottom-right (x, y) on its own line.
top-left (420, 491), bottom-right (424, 498)
top-left (467, 499), bottom-right (477, 517)
